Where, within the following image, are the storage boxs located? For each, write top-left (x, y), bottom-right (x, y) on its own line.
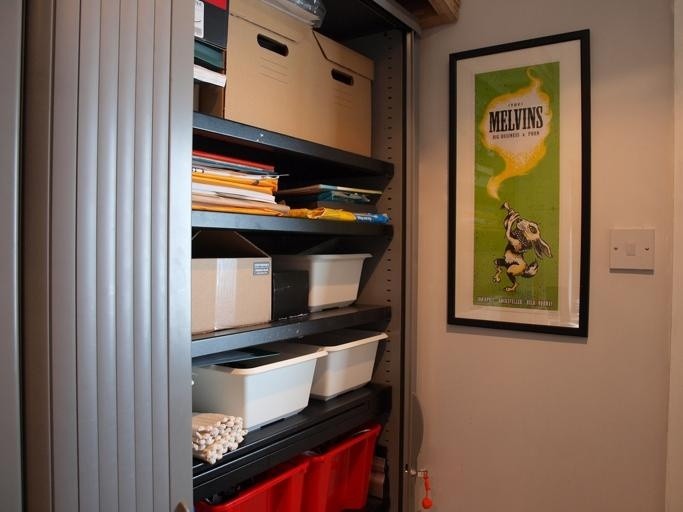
top-left (201, 1), bottom-right (374, 159)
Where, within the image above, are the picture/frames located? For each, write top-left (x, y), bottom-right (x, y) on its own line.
top-left (448, 28), bottom-right (589, 337)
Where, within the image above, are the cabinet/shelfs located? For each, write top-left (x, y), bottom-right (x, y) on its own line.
top-left (192, 0), bottom-right (420, 512)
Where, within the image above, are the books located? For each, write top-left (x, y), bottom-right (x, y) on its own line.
top-left (191, 149), bottom-right (383, 214)
